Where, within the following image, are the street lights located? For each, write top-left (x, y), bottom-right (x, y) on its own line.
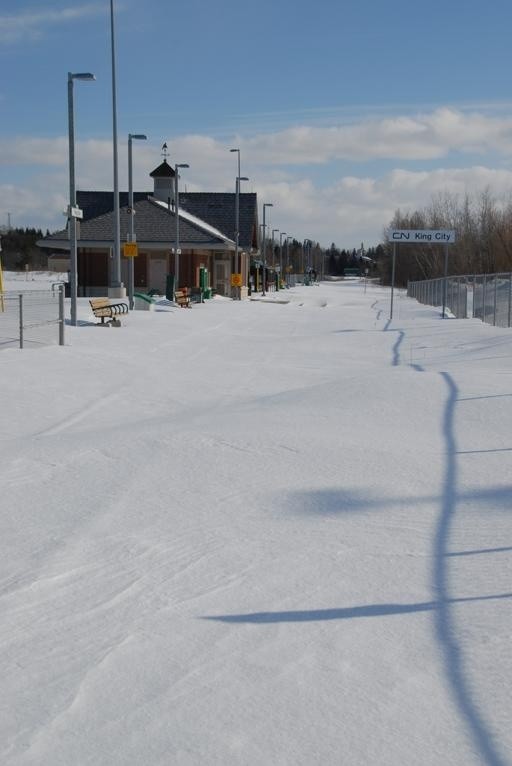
top-left (230, 148), bottom-right (249, 300)
top-left (173, 163), bottom-right (189, 303)
top-left (68, 72), bottom-right (96, 325)
top-left (260, 204), bottom-right (308, 296)
top-left (126, 134), bottom-right (148, 311)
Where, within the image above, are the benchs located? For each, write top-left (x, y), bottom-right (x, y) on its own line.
top-left (88, 297), bottom-right (129, 326)
top-left (174, 292), bottom-right (193, 309)
